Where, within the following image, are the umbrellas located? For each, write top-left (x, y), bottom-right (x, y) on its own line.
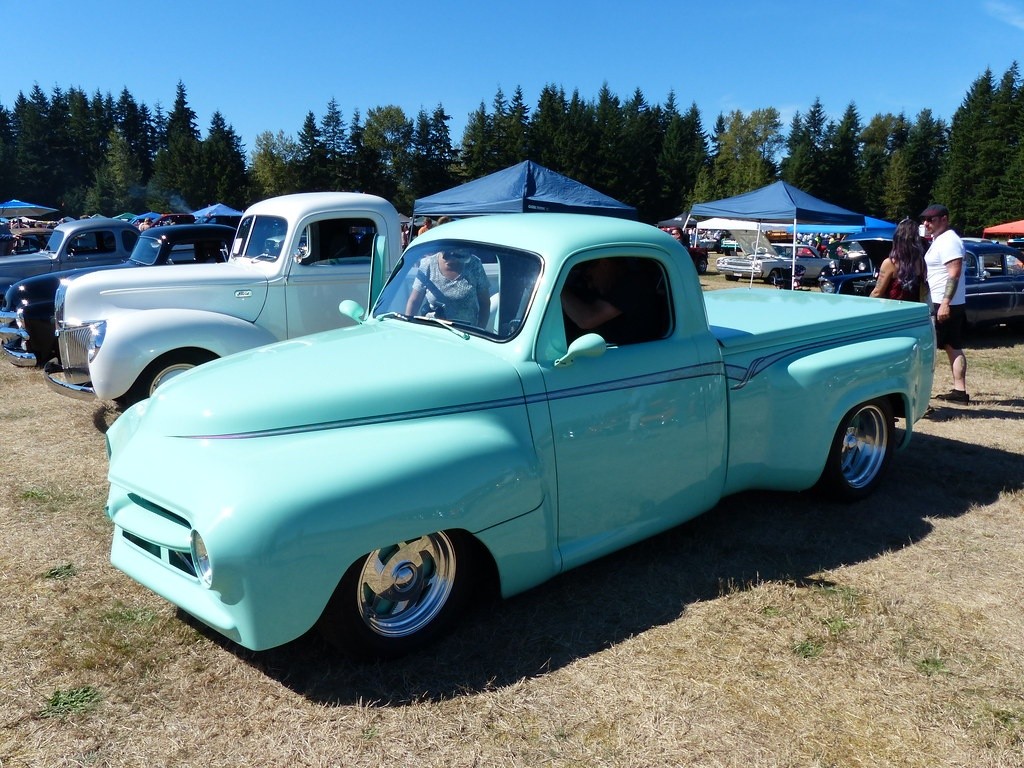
top-left (0, 196), bottom-right (243, 230)
top-left (983, 219), bottom-right (1024, 239)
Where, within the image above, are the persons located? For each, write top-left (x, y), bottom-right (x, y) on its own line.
top-left (137, 216), bottom-right (155, 231)
top-left (418, 217), bottom-right (430, 236)
top-left (11, 218), bottom-right (29, 255)
top-left (437, 215), bottom-right (451, 226)
top-left (869, 219), bottom-right (922, 301)
top-left (399, 226), bottom-right (408, 250)
top-left (671, 227), bottom-right (683, 243)
top-left (920, 204), bottom-right (968, 406)
top-left (46, 222), bottom-right (54, 228)
top-left (57, 216), bottom-right (66, 222)
top-left (560, 260), bottom-right (636, 345)
top-left (405, 246), bottom-right (491, 329)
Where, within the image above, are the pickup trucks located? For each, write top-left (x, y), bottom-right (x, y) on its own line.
top-left (103, 214), bottom-right (937, 652)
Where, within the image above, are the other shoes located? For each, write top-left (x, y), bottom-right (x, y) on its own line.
top-left (923, 406), bottom-right (935, 418)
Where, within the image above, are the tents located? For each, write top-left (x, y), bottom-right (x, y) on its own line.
top-left (683, 180), bottom-right (896, 291)
top-left (406, 159), bottom-right (636, 250)
top-left (658, 211), bottom-right (697, 227)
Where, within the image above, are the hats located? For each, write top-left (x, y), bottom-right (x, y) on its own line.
top-left (919, 204), bottom-right (949, 218)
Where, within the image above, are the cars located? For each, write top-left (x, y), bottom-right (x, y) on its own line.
top-left (44, 192), bottom-right (499, 410)
top-left (818, 229), bottom-right (1024, 349)
top-left (0, 224), bottom-right (237, 367)
top-left (715, 230), bottom-right (839, 285)
top-left (0, 217), bottom-right (194, 309)
top-left (10, 228), bottom-right (53, 254)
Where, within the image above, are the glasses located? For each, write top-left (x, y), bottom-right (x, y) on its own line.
top-left (922, 217), bottom-right (937, 222)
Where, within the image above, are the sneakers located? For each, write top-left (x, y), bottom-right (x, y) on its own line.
top-left (936, 389), bottom-right (970, 405)
